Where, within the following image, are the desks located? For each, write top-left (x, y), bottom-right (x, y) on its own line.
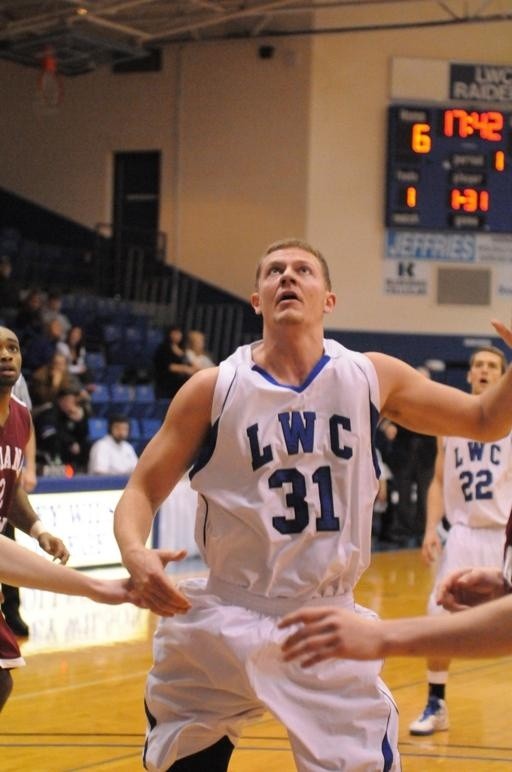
top-left (15, 475), bottom-right (157, 569)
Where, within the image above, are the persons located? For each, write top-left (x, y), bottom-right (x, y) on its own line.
top-left (407, 336), bottom-right (512, 738)
top-left (0, 533), bottom-right (146, 609)
top-left (0, 324), bottom-right (75, 705)
top-left (109, 237), bottom-right (511, 772)
top-left (0, 253), bottom-right (139, 475)
top-left (154, 328), bottom-right (216, 400)
top-left (275, 560), bottom-right (511, 676)
top-left (374, 367), bottom-right (438, 547)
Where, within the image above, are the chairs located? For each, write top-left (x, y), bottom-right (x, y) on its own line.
top-left (85, 302), bottom-right (173, 455)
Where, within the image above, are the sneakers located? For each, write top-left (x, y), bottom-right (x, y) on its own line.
top-left (409, 695), bottom-right (449, 736)
